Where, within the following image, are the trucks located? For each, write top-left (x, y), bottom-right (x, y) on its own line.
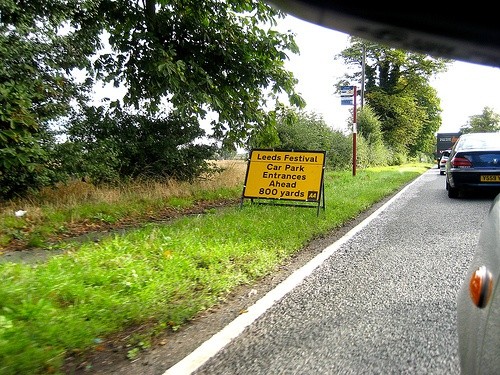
top-left (435, 133), bottom-right (463, 169)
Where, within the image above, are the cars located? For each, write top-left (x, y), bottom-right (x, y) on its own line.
top-left (438, 150), bottom-right (451, 175)
top-left (441, 132), bottom-right (500, 200)
